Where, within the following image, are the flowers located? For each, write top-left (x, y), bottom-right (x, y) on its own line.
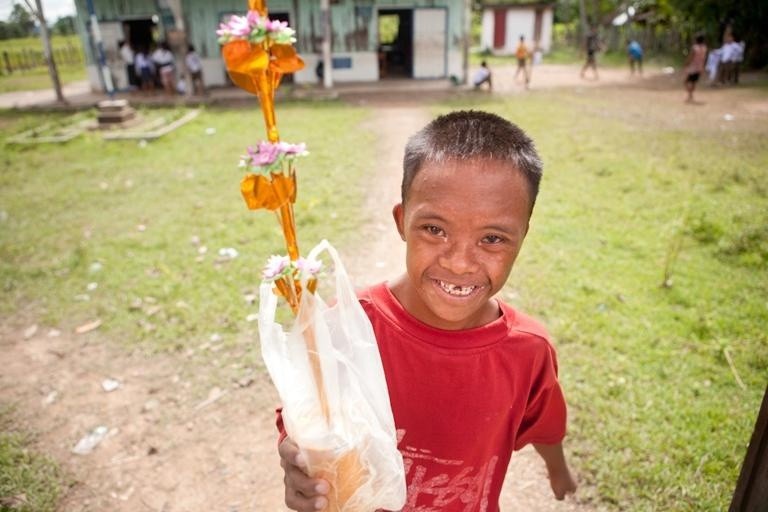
top-left (237, 141), bottom-right (306, 180)
top-left (260, 249), bottom-right (321, 281)
top-left (217, 12), bottom-right (298, 52)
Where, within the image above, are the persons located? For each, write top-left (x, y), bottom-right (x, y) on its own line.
top-left (580, 23), bottom-right (602, 79)
top-left (513, 35), bottom-right (531, 79)
top-left (276, 110), bottom-right (579, 512)
top-left (626, 38), bottom-right (643, 75)
top-left (119, 40), bottom-right (205, 98)
top-left (678, 32), bottom-right (744, 104)
top-left (472, 61), bottom-right (491, 88)
